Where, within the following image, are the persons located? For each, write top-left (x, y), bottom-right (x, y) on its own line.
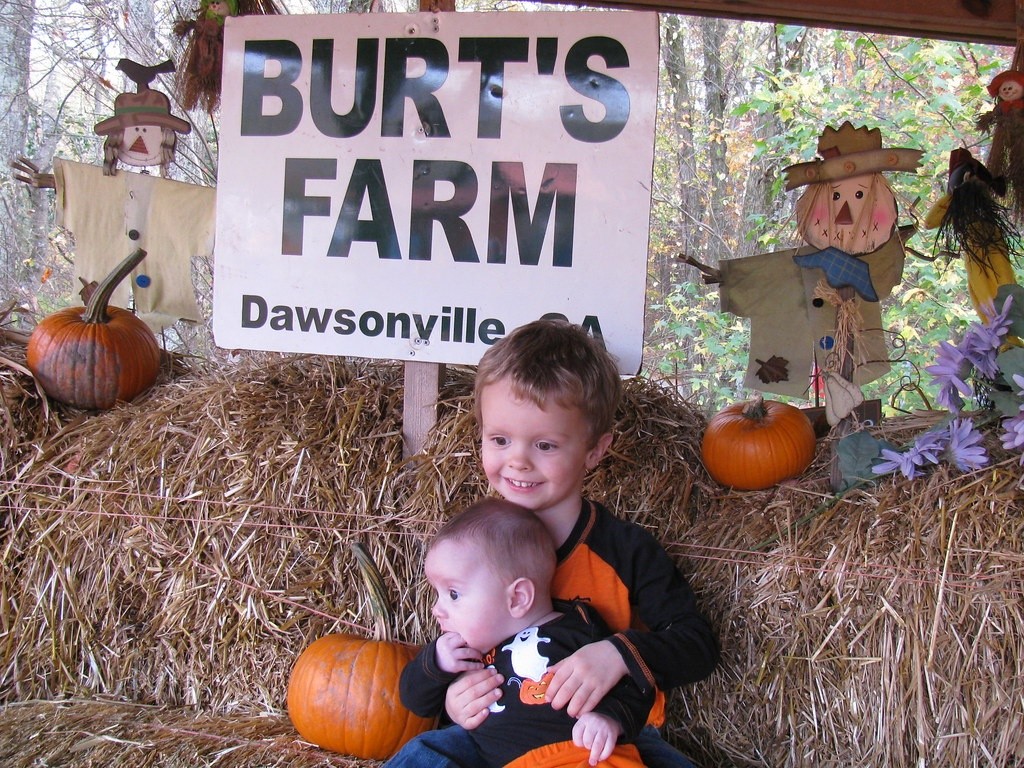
top-left (400, 497), bottom-right (657, 768)
top-left (381, 320), bottom-right (721, 768)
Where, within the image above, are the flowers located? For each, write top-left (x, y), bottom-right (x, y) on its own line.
top-left (867, 292), bottom-right (1023, 495)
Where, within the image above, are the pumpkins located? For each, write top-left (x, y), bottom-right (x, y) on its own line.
top-left (26, 246), bottom-right (160, 409)
top-left (287, 542), bottom-right (439, 760)
top-left (696, 395), bottom-right (817, 490)
